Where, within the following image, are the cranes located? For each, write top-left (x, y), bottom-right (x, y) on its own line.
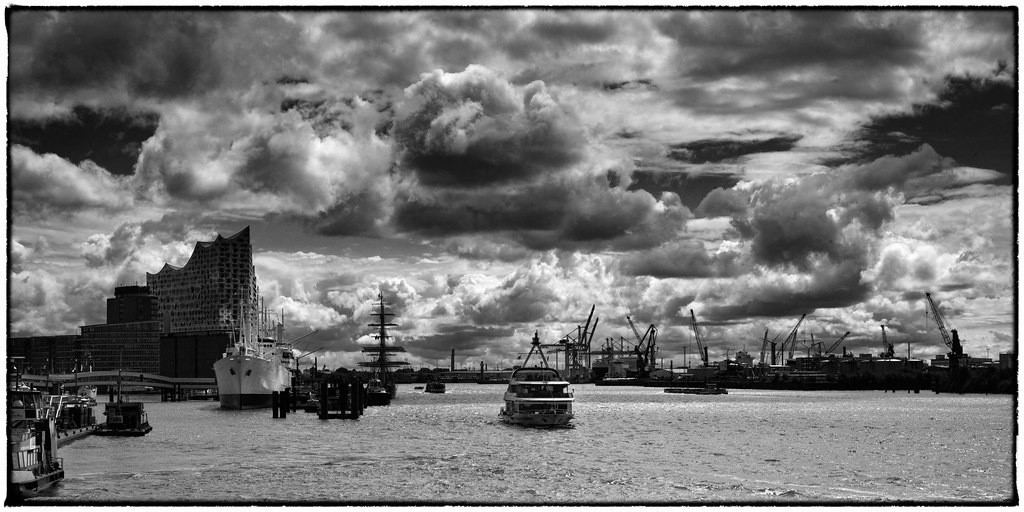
top-left (536, 292), bottom-right (993, 386)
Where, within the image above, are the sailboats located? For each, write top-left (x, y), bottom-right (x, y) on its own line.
top-left (354, 290), bottom-right (411, 399)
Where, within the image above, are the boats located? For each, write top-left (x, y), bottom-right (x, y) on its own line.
top-left (497, 329), bottom-right (576, 426)
top-left (364, 377), bottom-right (391, 406)
top-left (8, 354), bottom-right (153, 498)
top-left (425, 380), bottom-right (448, 393)
top-left (212, 279), bottom-right (320, 412)
